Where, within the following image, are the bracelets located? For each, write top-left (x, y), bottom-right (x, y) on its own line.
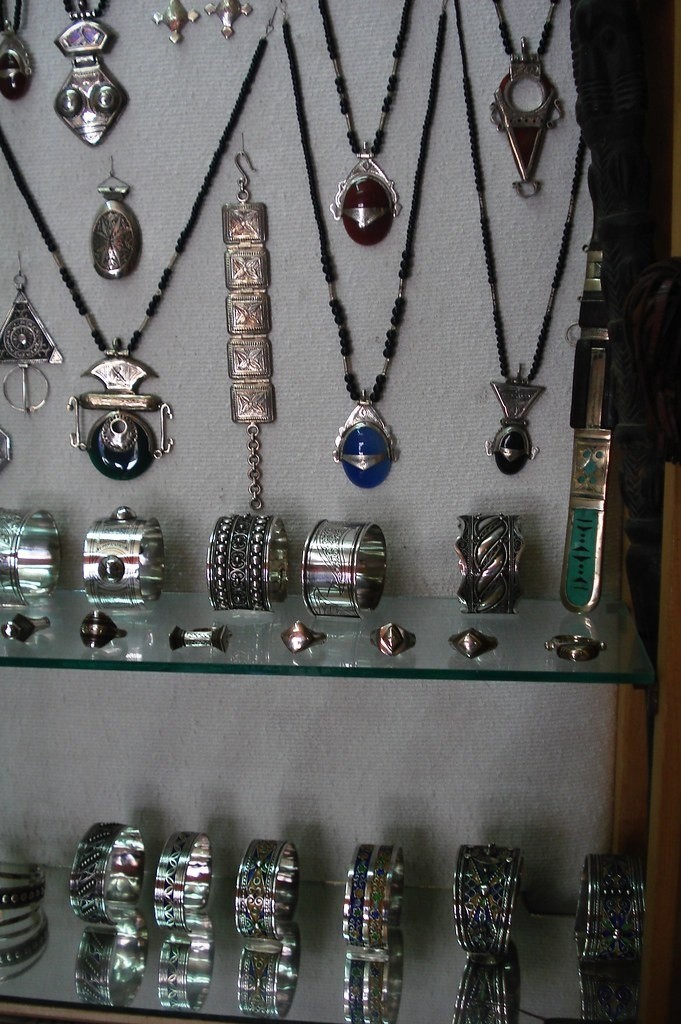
top-left (1, 907), bottom-right (639, 1024)
top-left (0, 507), bottom-right (525, 620)
top-left (0, 822), bottom-right (642, 957)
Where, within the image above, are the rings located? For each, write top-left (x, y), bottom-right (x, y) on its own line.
top-left (8, 608), bottom-right (606, 662)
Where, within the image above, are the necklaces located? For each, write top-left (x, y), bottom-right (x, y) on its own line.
top-left (0, 0), bottom-right (592, 481)
